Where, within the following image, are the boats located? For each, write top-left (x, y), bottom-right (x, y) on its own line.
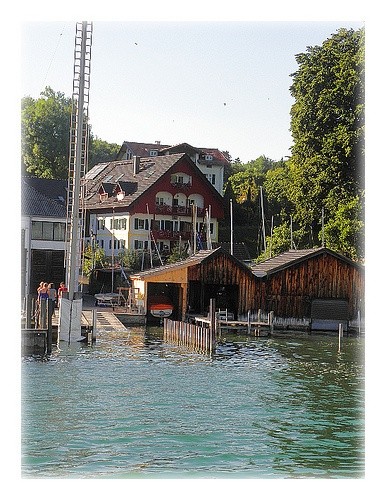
top-left (149, 294), bottom-right (175, 319)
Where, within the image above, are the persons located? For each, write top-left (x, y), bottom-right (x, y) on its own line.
top-left (47, 283), bottom-right (57, 318)
top-left (38, 283), bottom-right (50, 303)
top-left (57, 282), bottom-right (68, 308)
top-left (37, 282), bottom-right (45, 291)
